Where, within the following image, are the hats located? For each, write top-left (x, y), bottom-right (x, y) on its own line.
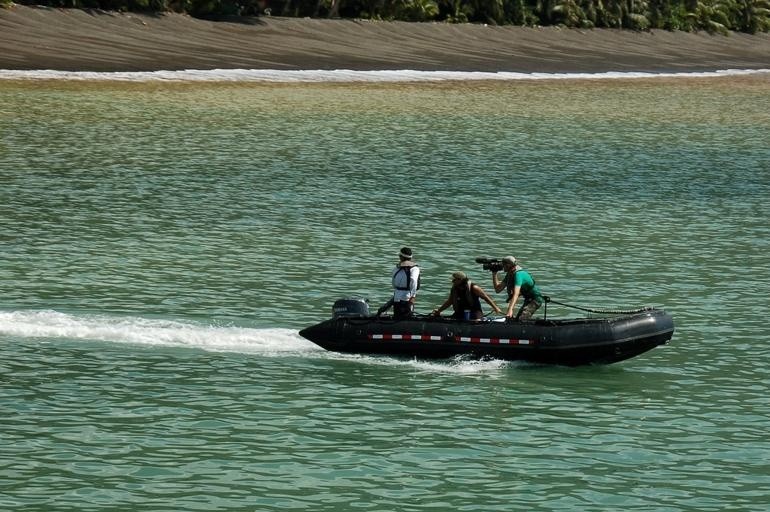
top-left (448, 272), bottom-right (468, 282)
top-left (501, 256), bottom-right (518, 267)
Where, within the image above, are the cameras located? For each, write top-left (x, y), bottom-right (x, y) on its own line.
top-left (476, 257), bottom-right (503, 272)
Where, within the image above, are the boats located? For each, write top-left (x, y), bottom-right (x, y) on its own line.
top-left (297, 297), bottom-right (676, 368)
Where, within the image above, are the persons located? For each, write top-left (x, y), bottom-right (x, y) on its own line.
top-left (431, 271), bottom-right (502, 319)
top-left (390, 247), bottom-right (420, 315)
top-left (492, 255), bottom-right (544, 319)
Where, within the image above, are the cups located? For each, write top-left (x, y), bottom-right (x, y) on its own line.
top-left (464, 310), bottom-right (471, 319)
